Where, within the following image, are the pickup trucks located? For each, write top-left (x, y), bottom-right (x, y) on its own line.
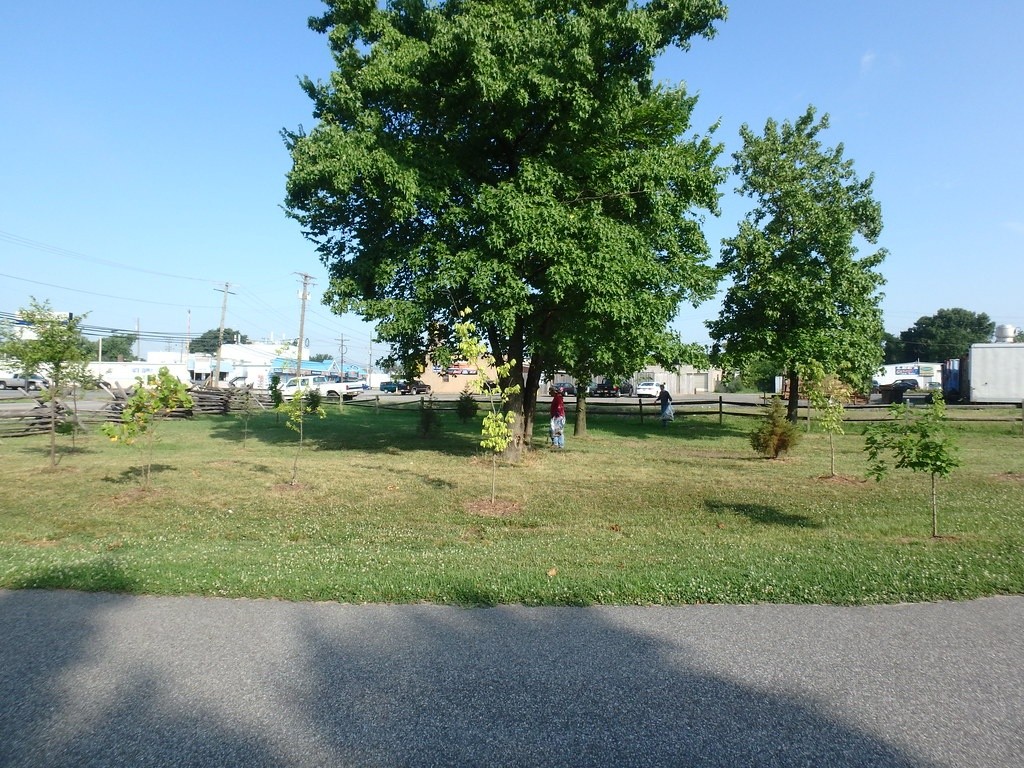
top-left (0, 374), bottom-right (50, 391)
top-left (597, 377), bottom-right (634, 399)
top-left (269, 376), bottom-right (363, 401)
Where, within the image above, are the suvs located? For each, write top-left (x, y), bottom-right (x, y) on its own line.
top-left (400, 380), bottom-right (432, 395)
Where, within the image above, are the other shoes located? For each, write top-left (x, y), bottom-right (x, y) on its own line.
top-left (548, 445), bottom-right (557, 449)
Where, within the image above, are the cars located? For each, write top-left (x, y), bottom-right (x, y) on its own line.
top-left (548, 382), bottom-right (575, 397)
top-left (573, 382), bottom-right (599, 397)
top-left (637, 382), bottom-right (666, 398)
top-left (380, 382), bottom-right (402, 393)
top-left (925, 382), bottom-right (942, 392)
top-left (871, 380), bottom-right (881, 394)
top-left (889, 378), bottom-right (918, 394)
top-left (81, 379), bottom-right (111, 390)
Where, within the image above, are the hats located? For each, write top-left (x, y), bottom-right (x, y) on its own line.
top-left (549, 387), bottom-right (558, 391)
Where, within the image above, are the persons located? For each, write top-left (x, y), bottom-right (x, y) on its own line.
top-left (663, 382), bottom-right (667, 390)
top-left (549, 388), bottom-right (566, 449)
top-left (655, 385), bottom-right (673, 427)
